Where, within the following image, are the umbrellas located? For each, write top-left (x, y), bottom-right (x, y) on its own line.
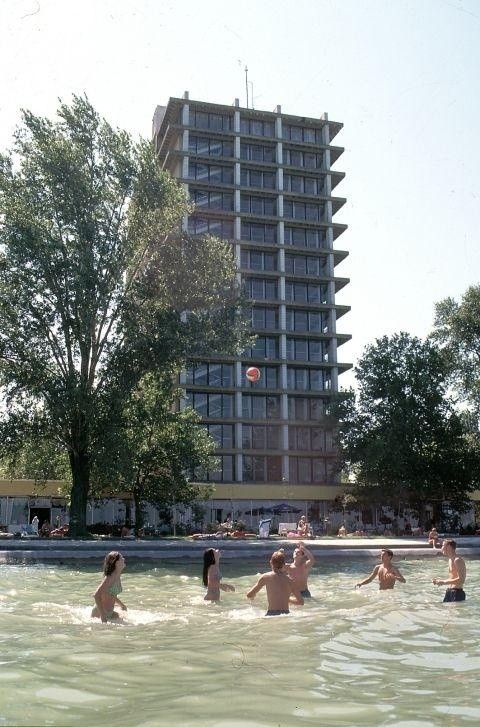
top-left (246, 502), bottom-right (303, 523)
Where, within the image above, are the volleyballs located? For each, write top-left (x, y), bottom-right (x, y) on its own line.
top-left (246, 367), bottom-right (261, 382)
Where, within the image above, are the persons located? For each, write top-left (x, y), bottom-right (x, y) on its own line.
top-left (428, 528), bottom-right (439, 549)
top-left (355, 549), bottom-right (406, 590)
top-left (92, 551), bottom-right (127, 622)
top-left (203, 540), bottom-right (316, 616)
top-left (120, 523), bottom-right (131, 538)
top-left (432, 539), bottom-right (466, 602)
top-left (297, 519), bottom-right (309, 537)
top-left (355, 518), bottom-right (426, 536)
top-left (32, 516), bottom-right (70, 537)
top-left (232, 527), bottom-right (245, 536)
